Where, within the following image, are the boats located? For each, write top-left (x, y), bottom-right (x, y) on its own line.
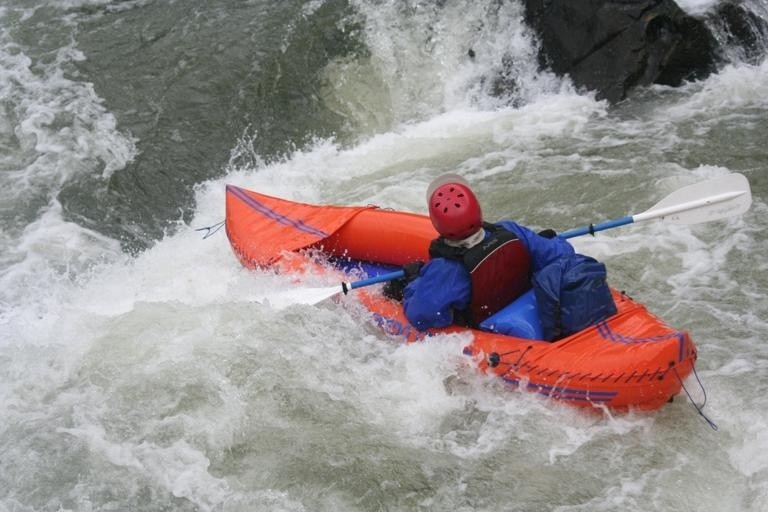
top-left (222, 182), bottom-right (702, 421)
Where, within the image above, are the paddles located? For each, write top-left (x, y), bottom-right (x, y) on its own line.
top-left (247, 173), bottom-right (752, 313)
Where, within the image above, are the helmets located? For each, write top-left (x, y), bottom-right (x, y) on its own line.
top-left (429, 183), bottom-right (483, 242)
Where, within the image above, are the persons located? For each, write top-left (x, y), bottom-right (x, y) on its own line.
top-left (389, 173), bottom-right (575, 339)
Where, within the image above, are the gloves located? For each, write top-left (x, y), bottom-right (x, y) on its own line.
top-left (538, 229), bottom-right (557, 239)
top-left (403, 261), bottom-right (424, 280)
